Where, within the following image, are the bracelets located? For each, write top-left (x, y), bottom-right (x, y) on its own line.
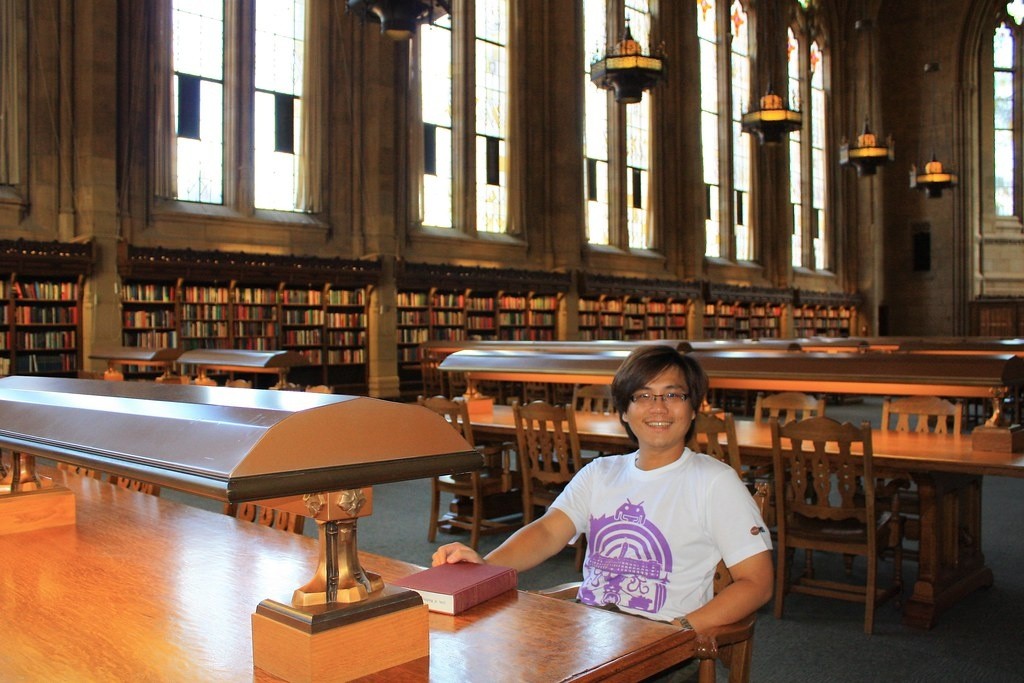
top-left (674, 616), bottom-right (692, 630)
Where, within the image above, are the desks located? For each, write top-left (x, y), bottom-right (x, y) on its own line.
top-left (465, 372), bottom-right (1013, 412)
top-left (441, 404), bottom-right (1024, 631)
top-left (0, 449), bottom-right (700, 683)
top-left (0, 463), bottom-right (702, 683)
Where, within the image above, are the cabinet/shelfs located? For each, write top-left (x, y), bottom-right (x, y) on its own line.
top-left (0, 238), bottom-right (864, 396)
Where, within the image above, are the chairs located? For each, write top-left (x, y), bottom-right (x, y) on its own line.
top-left (687, 411), bottom-right (774, 517)
top-left (222, 500), bottom-right (305, 535)
top-left (875, 393), bottom-right (967, 561)
top-left (509, 399), bottom-right (585, 573)
top-left (747, 392), bottom-right (829, 504)
top-left (526, 473), bottom-right (781, 683)
top-left (567, 382), bottom-right (616, 414)
top-left (104, 472), bottom-right (161, 496)
top-left (56, 460), bottom-right (102, 479)
top-left (762, 413), bottom-right (909, 637)
top-left (413, 394), bottom-right (522, 550)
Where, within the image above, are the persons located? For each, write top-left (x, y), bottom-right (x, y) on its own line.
top-left (432, 344), bottom-right (773, 683)
top-left (841, 328), bottom-right (849, 338)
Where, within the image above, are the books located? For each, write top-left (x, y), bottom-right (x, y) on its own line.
top-left (579, 299), bottom-right (686, 340)
top-left (794, 308), bottom-right (849, 337)
top-left (398, 293), bottom-right (557, 361)
top-left (391, 561), bottom-right (518, 615)
top-left (120, 285), bottom-right (367, 374)
top-left (704, 305), bottom-right (780, 338)
top-left (0, 280), bottom-right (79, 375)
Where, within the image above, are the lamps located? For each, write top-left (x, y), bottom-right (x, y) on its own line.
top-left (838, 19), bottom-right (896, 178)
top-left (434, 344), bottom-right (1024, 453)
top-left (907, 58), bottom-right (960, 198)
top-left (0, 370), bottom-right (489, 683)
top-left (589, 15), bottom-right (668, 107)
top-left (803, 336), bottom-right (873, 351)
top-left (691, 335), bottom-right (806, 354)
top-left (889, 336), bottom-right (1024, 356)
top-left (427, 338), bottom-right (691, 398)
top-left (174, 347), bottom-right (311, 392)
top-left (87, 345), bottom-right (186, 384)
top-left (341, 0), bottom-right (434, 43)
top-left (740, 77), bottom-right (806, 148)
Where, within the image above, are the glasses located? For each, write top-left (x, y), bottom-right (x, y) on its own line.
top-left (629, 392), bottom-right (692, 407)
